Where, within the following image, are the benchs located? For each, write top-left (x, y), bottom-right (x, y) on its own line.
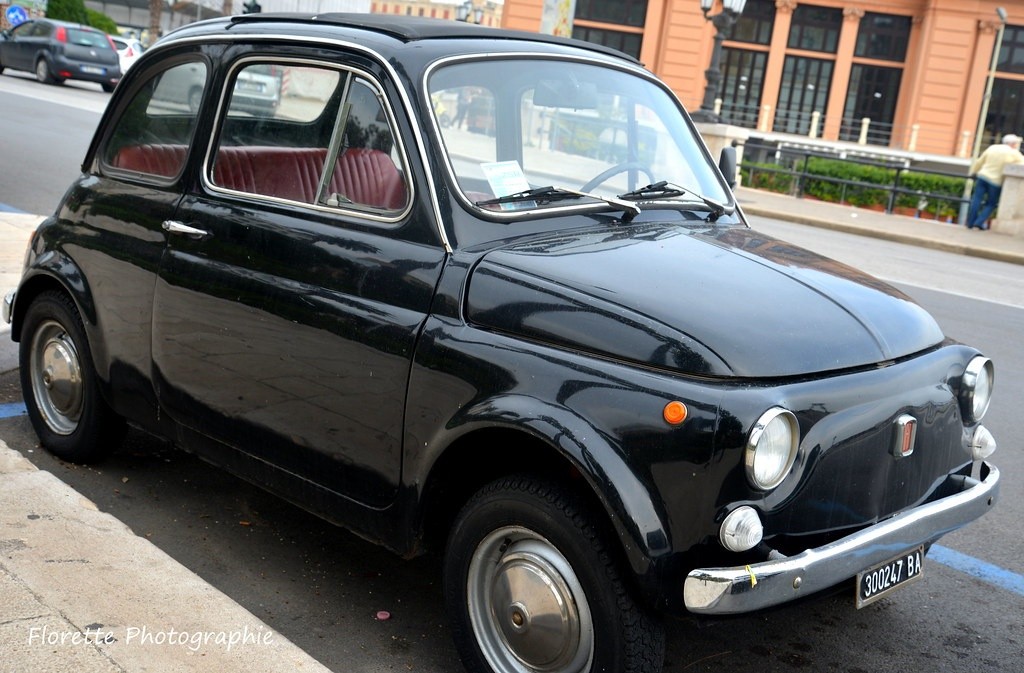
top-left (111, 143), bottom-right (408, 212)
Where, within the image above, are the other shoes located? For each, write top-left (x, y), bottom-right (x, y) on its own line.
top-left (973, 224), bottom-right (988, 230)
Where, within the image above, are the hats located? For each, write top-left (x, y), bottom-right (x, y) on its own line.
top-left (1001, 134), bottom-right (1023, 144)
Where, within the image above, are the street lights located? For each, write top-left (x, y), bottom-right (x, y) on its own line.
top-left (688, 0), bottom-right (749, 126)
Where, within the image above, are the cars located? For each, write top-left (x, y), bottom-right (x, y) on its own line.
top-left (2, 9), bottom-right (1002, 673)
top-left (150, 63), bottom-right (284, 118)
top-left (111, 36), bottom-right (145, 77)
top-left (0, 18), bottom-right (123, 93)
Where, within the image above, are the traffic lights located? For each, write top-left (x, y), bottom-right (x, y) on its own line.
top-left (239, 1), bottom-right (256, 14)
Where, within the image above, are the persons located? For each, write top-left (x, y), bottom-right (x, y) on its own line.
top-left (966, 134), bottom-right (1024, 230)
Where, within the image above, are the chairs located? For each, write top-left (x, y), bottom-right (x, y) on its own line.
top-left (463, 191), bottom-right (502, 214)
